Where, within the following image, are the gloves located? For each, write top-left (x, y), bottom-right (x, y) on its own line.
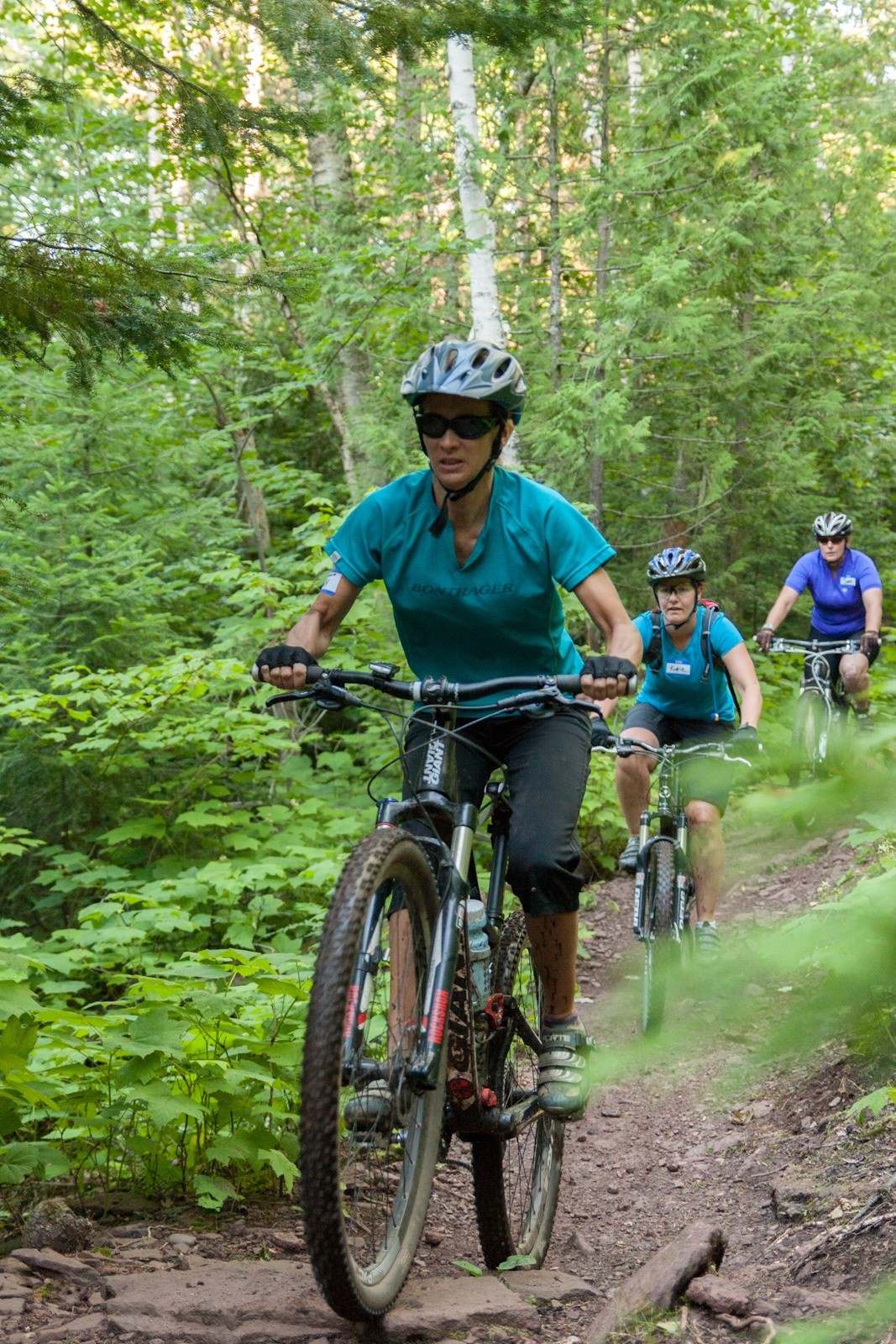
top-left (590, 716), bottom-right (611, 752)
top-left (728, 727), bottom-right (759, 759)
top-left (860, 630), bottom-right (879, 656)
top-left (754, 629), bottom-right (776, 654)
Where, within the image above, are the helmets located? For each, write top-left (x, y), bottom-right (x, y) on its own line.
top-left (397, 340), bottom-right (526, 424)
top-left (811, 511), bottom-right (854, 537)
top-left (645, 547), bottom-right (706, 586)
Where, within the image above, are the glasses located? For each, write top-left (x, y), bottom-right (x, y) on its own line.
top-left (414, 411), bottom-right (498, 440)
top-left (653, 583), bottom-right (694, 597)
top-left (817, 535), bottom-right (842, 542)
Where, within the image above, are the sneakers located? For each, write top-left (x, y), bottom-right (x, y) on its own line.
top-left (693, 921), bottom-right (722, 965)
top-left (538, 1014), bottom-right (592, 1114)
top-left (619, 835), bottom-right (642, 869)
top-left (856, 704), bottom-right (871, 735)
top-left (345, 1077), bottom-right (400, 1128)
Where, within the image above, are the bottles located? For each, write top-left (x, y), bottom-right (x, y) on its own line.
top-left (459, 900), bottom-right (492, 1012)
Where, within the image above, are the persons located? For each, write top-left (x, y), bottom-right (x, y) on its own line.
top-left (254, 340), bottom-right (645, 1134)
top-left (757, 511), bottom-right (883, 732)
top-left (589, 547), bottom-right (763, 956)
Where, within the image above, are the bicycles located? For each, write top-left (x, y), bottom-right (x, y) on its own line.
top-left (752, 635), bottom-right (883, 788)
top-left (590, 734), bottom-right (763, 1038)
top-left (250, 659), bottom-right (638, 1325)
